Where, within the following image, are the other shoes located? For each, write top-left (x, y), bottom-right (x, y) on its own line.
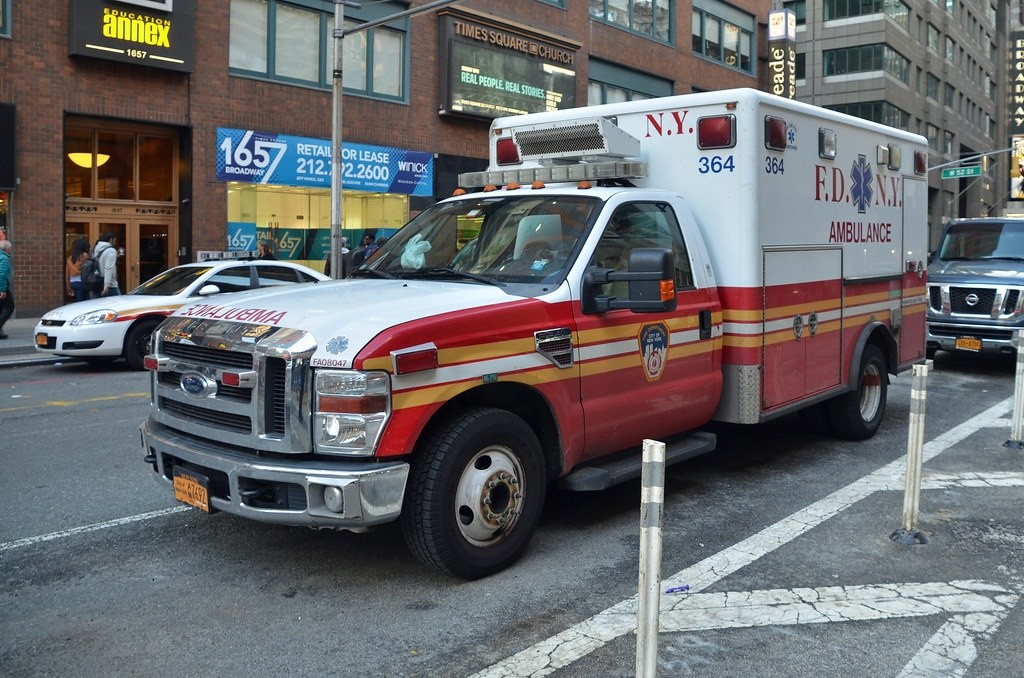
top-left (0, 332), bottom-right (8, 339)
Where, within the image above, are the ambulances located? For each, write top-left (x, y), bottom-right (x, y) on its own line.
top-left (137, 83), bottom-right (930, 580)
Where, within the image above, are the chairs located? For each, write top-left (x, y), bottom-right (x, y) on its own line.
top-left (496, 241), bottom-right (552, 278)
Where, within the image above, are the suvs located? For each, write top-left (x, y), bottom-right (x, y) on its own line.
top-left (927, 216), bottom-right (1024, 366)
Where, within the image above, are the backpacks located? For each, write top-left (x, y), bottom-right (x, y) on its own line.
top-left (81, 246), bottom-right (115, 290)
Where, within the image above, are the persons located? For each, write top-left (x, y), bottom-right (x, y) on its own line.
top-left (256, 240), bottom-right (276, 261)
top-left (342, 234), bottom-right (386, 267)
top-left (66, 239), bottom-right (90, 303)
top-left (0, 240), bottom-right (14, 340)
top-left (89, 230), bottom-right (121, 301)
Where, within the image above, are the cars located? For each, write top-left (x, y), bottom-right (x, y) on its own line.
top-left (33, 252), bottom-right (336, 374)
